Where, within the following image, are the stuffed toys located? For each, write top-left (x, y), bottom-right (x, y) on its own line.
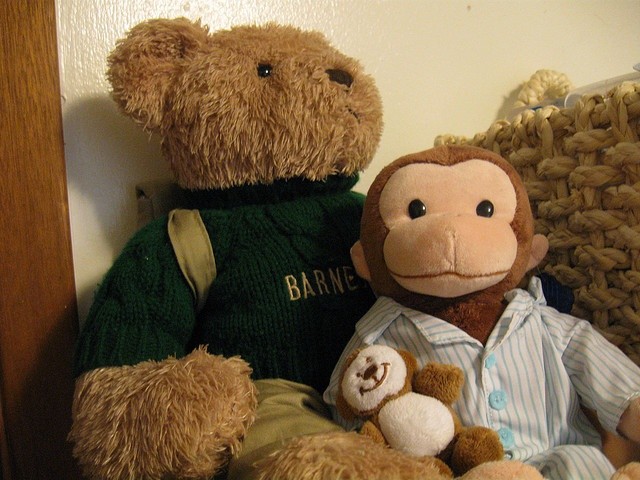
top-left (322, 146), bottom-right (639, 480)
top-left (339, 341), bottom-right (503, 473)
top-left (73, 16), bottom-right (481, 479)
